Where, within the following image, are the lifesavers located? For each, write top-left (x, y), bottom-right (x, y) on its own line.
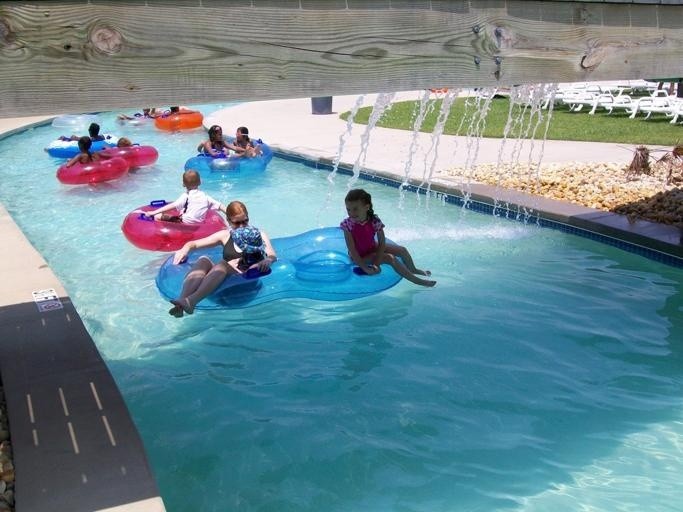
top-left (155, 108), bottom-right (203, 129)
top-left (113, 112), bottom-right (165, 130)
top-left (56, 156), bottom-right (128, 184)
top-left (104, 146), bottom-right (158, 167)
top-left (155, 228), bottom-right (403, 311)
top-left (43, 134), bottom-right (118, 157)
top-left (122, 201), bottom-right (224, 249)
top-left (185, 139), bottom-right (272, 178)
top-left (52, 114), bottom-right (97, 131)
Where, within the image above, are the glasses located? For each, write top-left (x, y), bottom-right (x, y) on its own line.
top-left (230, 216), bottom-right (248, 224)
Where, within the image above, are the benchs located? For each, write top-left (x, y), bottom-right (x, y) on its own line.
top-left (555, 80), bottom-right (683, 125)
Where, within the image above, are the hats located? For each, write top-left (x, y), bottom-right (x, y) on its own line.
top-left (232, 227), bottom-right (265, 253)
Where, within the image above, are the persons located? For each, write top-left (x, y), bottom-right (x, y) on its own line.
top-left (57, 122), bottom-right (105, 143)
top-left (225, 126), bottom-right (261, 158)
top-left (66, 136), bottom-right (102, 167)
top-left (340, 189), bottom-right (437, 288)
top-left (104, 137), bottom-right (134, 151)
top-left (199, 124), bottom-right (261, 158)
top-left (130, 169), bottom-right (227, 225)
top-left (228, 226), bottom-right (266, 275)
top-left (168, 200), bottom-right (278, 319)
top-left (116, 107), bottom-right (164, 122)
top-left (150, 105), bottom-right (203, 121)
